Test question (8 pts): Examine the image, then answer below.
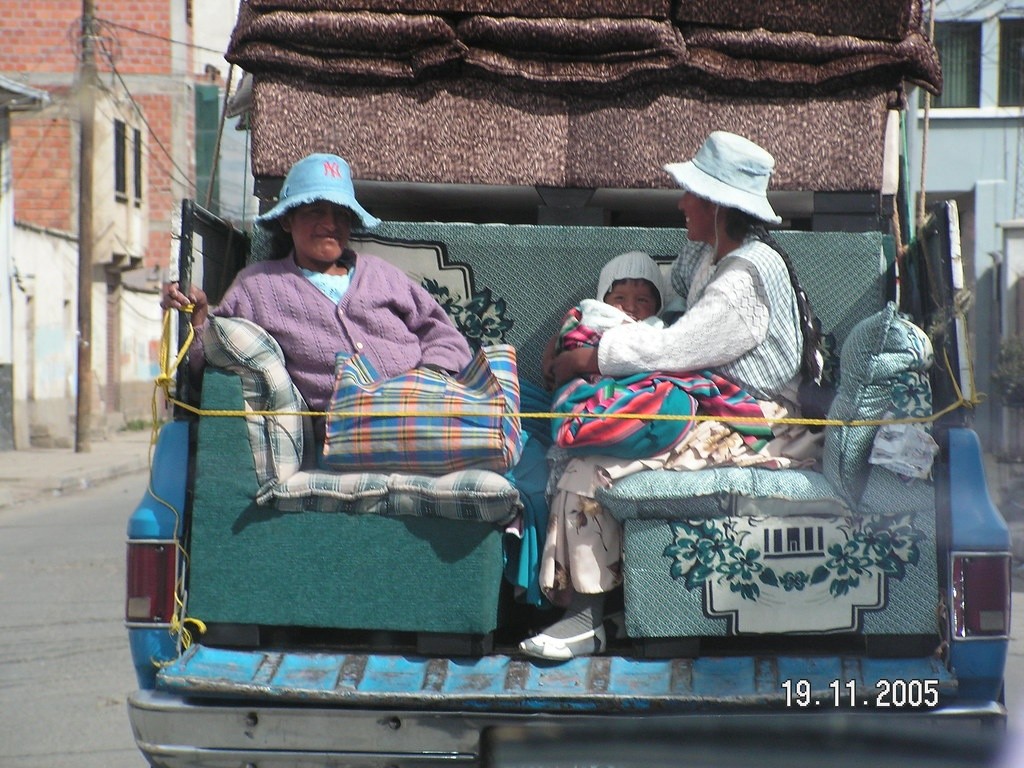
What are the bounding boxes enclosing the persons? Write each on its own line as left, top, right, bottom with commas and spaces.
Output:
160, 152, 567, 623
519, 130, 838, 661
550, 250, 699, 461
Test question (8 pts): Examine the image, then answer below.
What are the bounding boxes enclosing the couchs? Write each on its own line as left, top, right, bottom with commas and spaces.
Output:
592, 300, 943, 658
183, 311, 527, 657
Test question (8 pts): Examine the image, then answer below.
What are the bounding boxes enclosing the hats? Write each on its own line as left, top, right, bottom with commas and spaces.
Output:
663, 129, 784, 225
253, 153, 381, 233
596, 248, 668, 318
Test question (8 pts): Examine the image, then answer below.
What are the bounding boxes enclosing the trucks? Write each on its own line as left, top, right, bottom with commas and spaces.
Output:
125, 0, 1009, 768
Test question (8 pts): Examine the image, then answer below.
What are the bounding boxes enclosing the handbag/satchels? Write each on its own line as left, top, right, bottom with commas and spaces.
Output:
322, 343, 522, 476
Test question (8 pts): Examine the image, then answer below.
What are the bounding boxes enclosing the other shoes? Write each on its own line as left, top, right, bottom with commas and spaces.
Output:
527, 609, 629, 644
519, 625, 604, 661
